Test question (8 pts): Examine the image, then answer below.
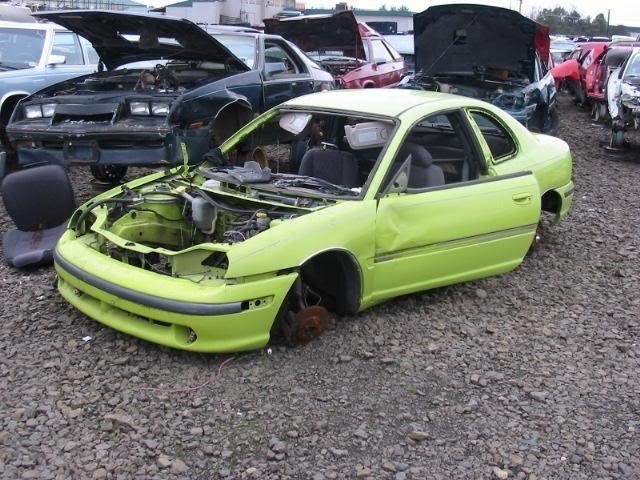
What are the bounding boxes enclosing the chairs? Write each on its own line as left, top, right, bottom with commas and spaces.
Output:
379, 141, 445, 193
2, 163, 78, 269
297, 148, 358, 190
